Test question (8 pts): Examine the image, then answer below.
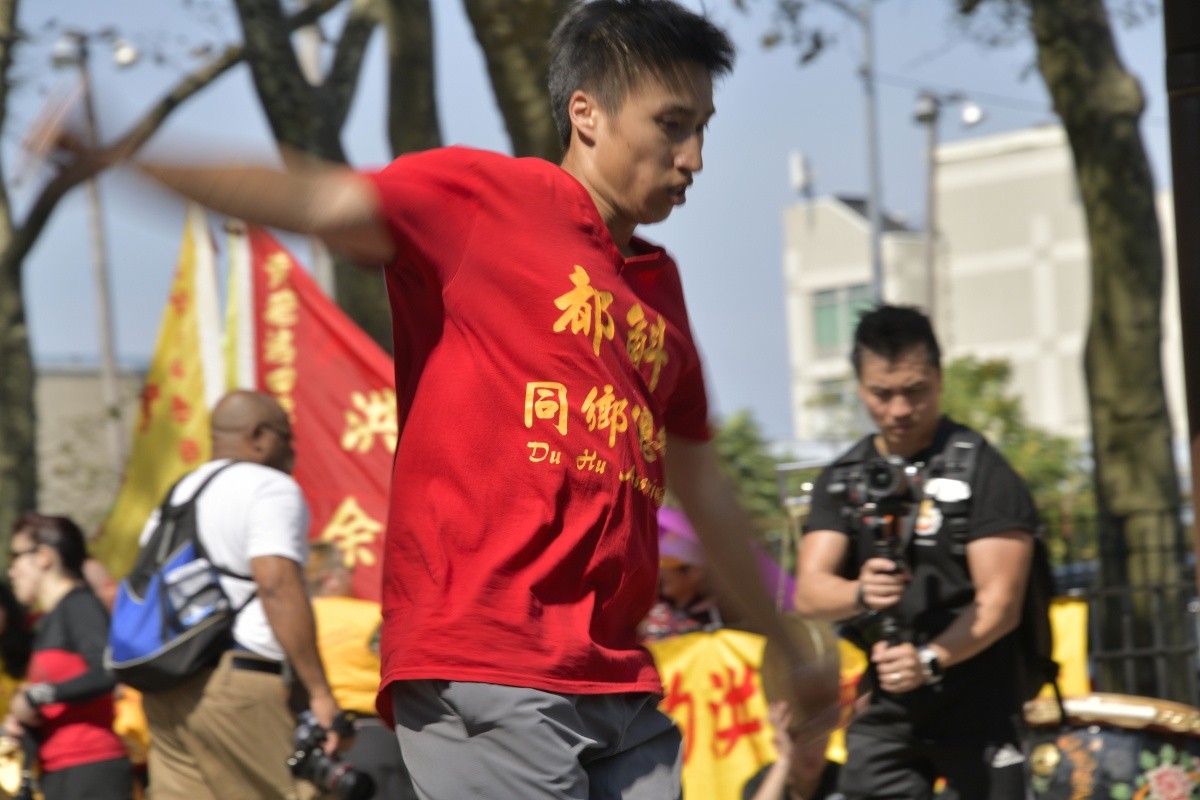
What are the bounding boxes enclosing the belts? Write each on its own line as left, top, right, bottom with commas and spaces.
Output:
204, 653, 283, 674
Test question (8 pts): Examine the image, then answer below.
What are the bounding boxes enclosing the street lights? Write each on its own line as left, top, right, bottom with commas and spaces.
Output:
910, 87, 985, 334
43, 26, 145, 477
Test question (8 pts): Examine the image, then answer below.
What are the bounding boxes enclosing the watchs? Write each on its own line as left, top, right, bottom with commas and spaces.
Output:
914, 644, 945, 687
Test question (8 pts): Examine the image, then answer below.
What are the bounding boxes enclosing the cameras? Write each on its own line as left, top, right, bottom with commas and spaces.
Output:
287, 723, 378, 800
846, 457, 922, 507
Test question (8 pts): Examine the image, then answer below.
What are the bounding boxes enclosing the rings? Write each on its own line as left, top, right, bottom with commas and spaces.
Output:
893, 673, 900, 682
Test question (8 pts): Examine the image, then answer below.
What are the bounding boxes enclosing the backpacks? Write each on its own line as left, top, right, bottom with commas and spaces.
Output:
102, 461, 256, 691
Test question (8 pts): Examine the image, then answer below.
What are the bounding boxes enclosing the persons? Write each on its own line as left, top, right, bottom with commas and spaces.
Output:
792, 307, 1043, 800
1, 516, 133, 800
128, 391, 356, 798
26, 0, 840, 800
